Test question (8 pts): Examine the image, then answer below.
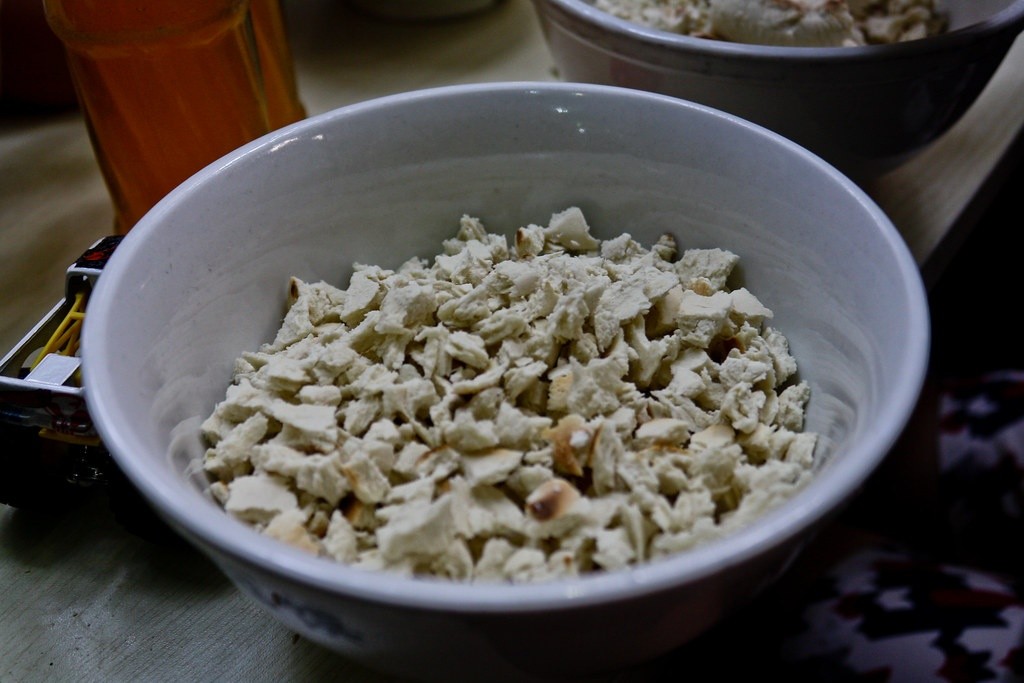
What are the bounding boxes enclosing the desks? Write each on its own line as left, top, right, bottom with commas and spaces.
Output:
0, 0, 1024, 683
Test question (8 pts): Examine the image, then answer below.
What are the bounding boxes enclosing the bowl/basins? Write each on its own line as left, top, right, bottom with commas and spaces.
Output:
523, 0, 1023, 178
82, 80, 930, 681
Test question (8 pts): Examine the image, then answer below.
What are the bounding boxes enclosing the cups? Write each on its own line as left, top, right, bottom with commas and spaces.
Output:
36, 0, 314, 268
